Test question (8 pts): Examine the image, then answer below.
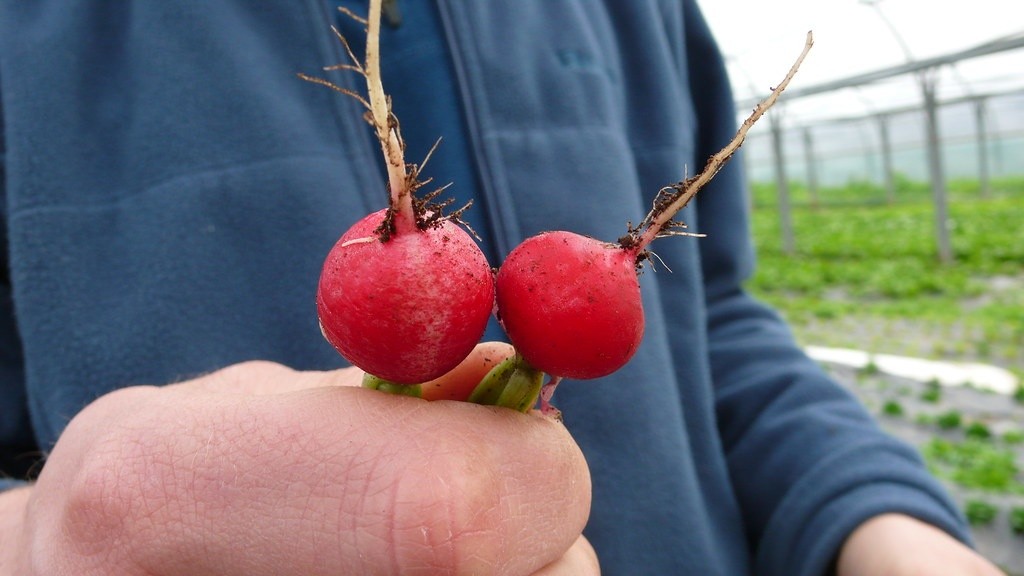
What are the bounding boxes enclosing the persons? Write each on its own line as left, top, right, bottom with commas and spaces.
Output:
1, 0, 1005, 576
